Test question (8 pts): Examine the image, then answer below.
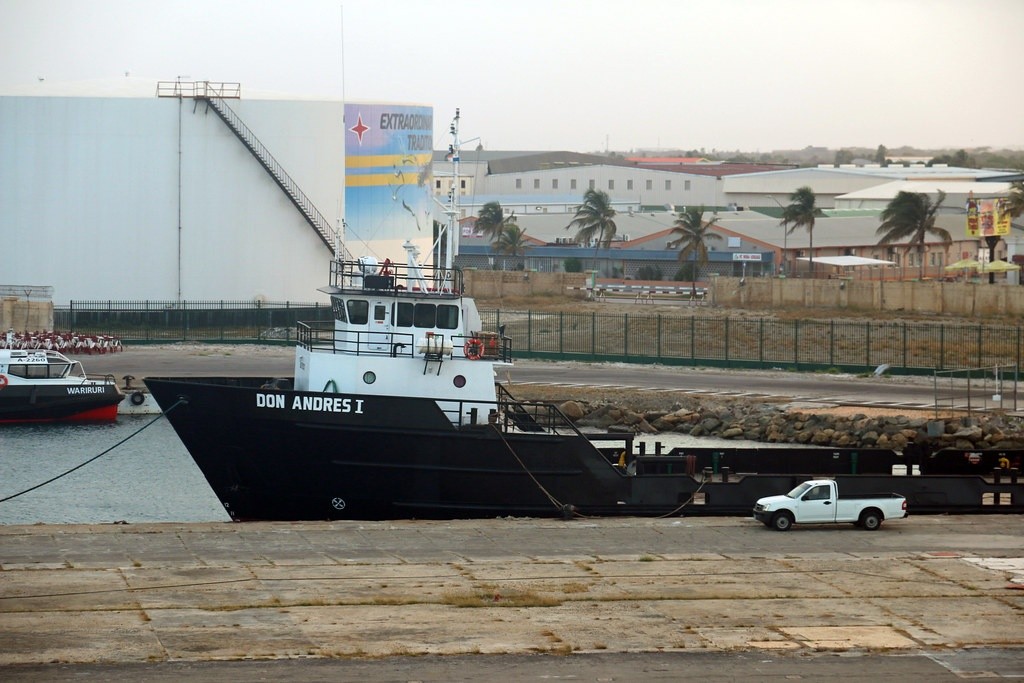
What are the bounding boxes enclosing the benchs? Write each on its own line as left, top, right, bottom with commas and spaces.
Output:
993, 467, 1018, 483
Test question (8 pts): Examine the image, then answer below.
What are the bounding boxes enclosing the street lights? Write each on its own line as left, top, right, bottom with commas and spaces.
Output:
766, 195, 787, 277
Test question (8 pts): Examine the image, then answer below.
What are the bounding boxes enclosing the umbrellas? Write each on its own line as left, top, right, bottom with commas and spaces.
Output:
977, 259, 1021, 285
946, 258, 980, 282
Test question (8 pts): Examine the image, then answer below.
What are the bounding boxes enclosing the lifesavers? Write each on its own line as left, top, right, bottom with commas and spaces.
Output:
0, 374, 8, 388
464, 339, 485, 360
131, 392, 144, 405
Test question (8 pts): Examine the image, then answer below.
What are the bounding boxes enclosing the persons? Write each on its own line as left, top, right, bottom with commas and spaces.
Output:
618, 451, 626, 473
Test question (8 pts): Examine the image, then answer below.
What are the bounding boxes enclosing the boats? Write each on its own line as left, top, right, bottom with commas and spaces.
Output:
0, 348, 126, 423
143, 103, 1024, 524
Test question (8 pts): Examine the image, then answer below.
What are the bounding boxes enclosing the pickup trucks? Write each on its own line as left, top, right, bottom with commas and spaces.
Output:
752, 479, 908, 532
